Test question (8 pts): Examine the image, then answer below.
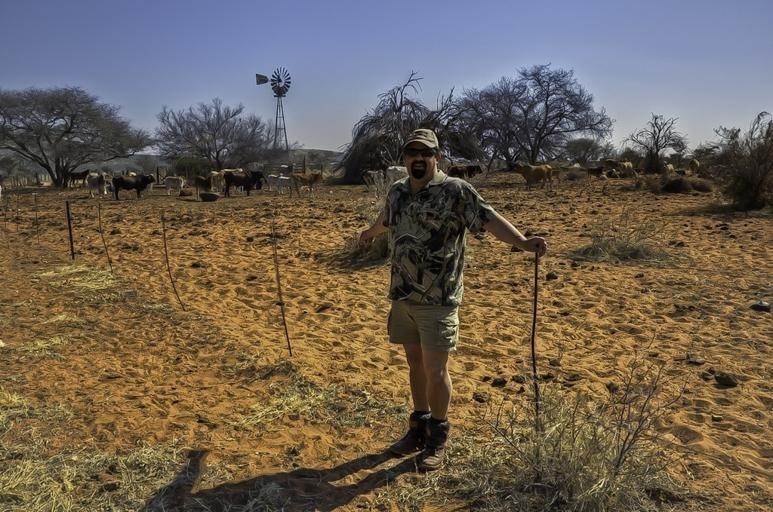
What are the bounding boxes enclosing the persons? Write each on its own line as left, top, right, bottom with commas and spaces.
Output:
356, 127, 549, 473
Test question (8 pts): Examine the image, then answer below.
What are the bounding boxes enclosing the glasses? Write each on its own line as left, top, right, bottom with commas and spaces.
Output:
404, 147, 437, 156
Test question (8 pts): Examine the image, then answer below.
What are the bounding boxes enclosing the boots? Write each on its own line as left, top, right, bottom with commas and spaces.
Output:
418, 421, 448, 470
390, 412, 431, 456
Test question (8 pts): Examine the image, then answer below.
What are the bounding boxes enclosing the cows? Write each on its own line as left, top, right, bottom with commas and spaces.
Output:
165, 176, 183, 196
664, 159, 699, 178
69, 169, 156, 200
360, 165, 409, 200
510, 160, 553, 192
193, 167, 263, 200
294, 167, 326, 200
588, 162, 649, 183
447, 165, 483, 181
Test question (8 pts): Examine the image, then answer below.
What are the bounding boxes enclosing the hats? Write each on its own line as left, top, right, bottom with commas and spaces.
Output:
403, 128, 440, 150
